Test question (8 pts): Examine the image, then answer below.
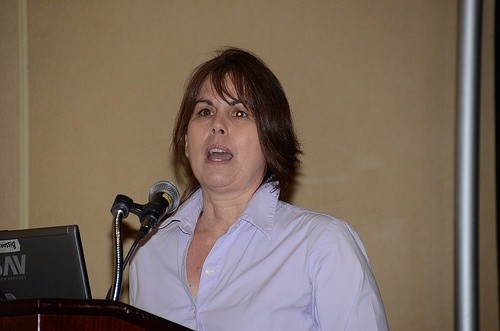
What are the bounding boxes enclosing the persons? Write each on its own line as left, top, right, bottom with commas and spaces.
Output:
129, 48, 390, 331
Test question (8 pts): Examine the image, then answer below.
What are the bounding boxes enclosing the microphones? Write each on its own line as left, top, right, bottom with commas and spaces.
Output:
139, 181, 180, 240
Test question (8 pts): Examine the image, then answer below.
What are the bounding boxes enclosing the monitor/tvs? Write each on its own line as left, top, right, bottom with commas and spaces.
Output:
0, 225, 92, 301
